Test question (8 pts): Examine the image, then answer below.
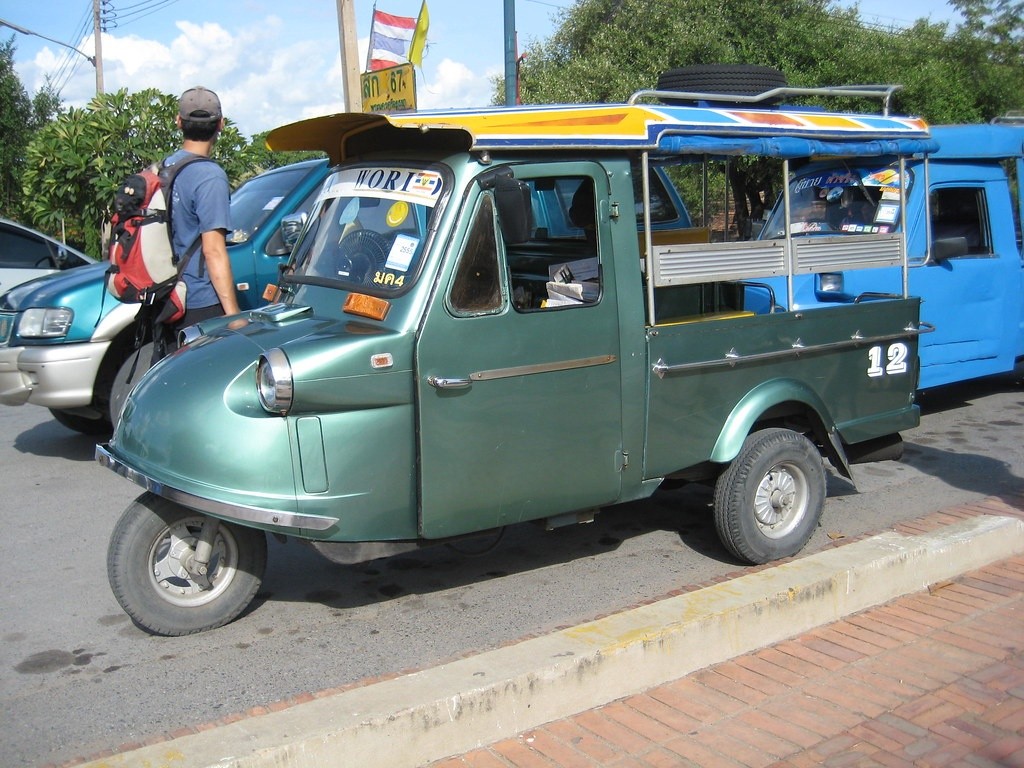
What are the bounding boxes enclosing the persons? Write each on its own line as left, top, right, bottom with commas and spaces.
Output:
152, 85, 249, 376
838, 187, 865, 224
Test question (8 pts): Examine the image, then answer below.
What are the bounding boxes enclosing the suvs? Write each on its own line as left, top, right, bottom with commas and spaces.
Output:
0, 157, 696, 434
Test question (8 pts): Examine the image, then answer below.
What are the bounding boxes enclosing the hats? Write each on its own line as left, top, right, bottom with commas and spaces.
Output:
180, 86, 222, 122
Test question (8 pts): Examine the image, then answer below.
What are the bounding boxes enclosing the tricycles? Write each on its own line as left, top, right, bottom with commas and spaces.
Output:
94, 65, 935, 636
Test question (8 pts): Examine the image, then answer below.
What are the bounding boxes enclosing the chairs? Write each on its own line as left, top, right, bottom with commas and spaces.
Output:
931, 201, 985, 257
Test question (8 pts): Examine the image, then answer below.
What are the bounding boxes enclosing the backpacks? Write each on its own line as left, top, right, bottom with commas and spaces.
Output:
108, 154, 216, 304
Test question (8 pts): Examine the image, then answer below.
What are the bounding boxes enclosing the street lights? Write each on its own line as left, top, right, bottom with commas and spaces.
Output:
0, 19, 104, 90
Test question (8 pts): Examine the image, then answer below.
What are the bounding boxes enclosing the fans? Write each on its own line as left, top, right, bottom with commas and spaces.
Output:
332, 229, 396, 290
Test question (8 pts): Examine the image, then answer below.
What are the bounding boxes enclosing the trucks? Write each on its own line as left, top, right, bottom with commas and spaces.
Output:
741, 117, 1024, 392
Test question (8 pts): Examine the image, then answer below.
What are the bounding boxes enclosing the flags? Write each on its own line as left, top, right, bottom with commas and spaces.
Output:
407, 0, 429, 68
369, 11, 417, 71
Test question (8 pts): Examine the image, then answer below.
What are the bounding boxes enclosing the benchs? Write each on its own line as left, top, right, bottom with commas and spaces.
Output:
636, 227, 785, 329
503, 244, 596, 296
